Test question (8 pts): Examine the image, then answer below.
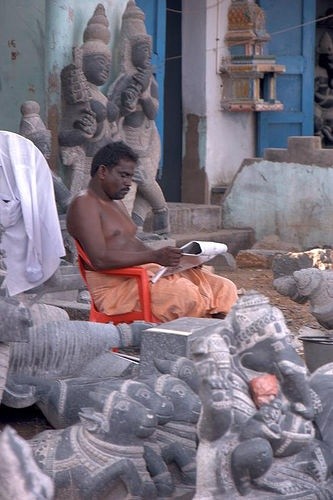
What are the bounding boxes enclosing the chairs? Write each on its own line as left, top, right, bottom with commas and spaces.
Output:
74, 239, 153, 324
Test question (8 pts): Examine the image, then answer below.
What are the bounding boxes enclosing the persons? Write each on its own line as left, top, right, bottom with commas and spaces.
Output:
66, 142, 240, 325
18, 1, 171, 265
